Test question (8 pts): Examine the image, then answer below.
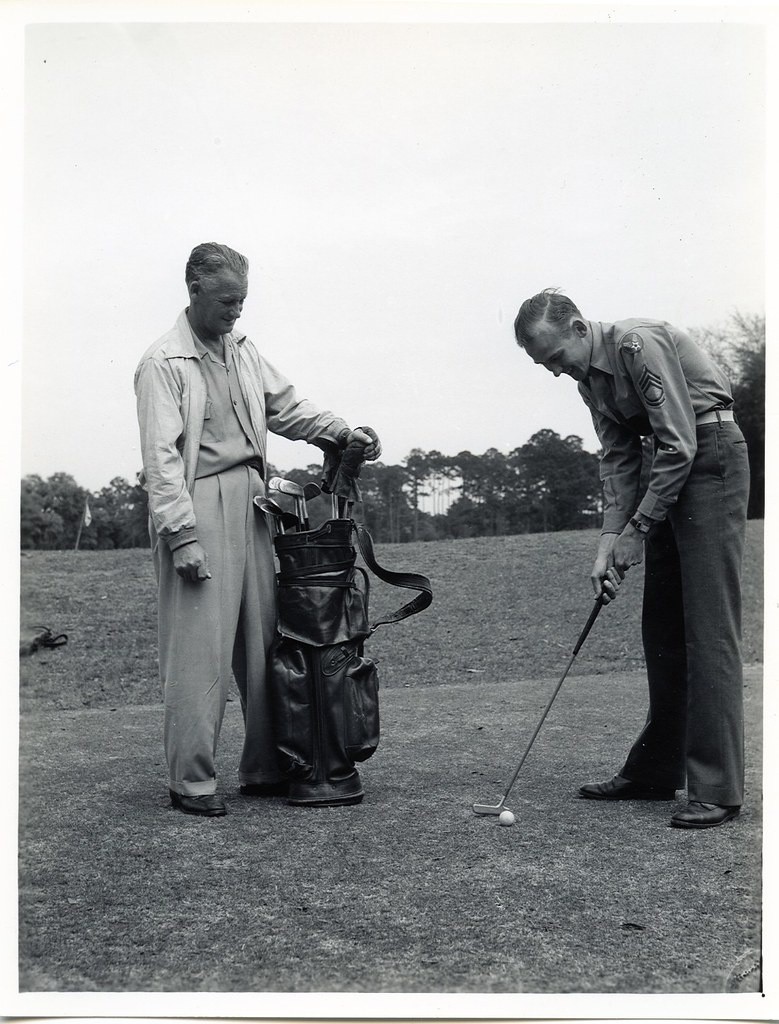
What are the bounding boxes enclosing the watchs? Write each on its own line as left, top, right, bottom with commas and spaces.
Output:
629, 518, 651, 534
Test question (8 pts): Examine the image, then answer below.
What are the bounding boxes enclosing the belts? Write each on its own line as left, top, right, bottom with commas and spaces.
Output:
695, 410, 733, 426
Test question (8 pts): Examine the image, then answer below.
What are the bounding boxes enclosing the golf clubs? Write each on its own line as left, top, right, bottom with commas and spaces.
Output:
472, 565, 618, 814
253, 434, 376, 538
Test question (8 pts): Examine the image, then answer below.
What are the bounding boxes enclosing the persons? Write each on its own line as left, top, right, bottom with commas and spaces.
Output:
133, 242, 381, 817
512, 290, 751, 831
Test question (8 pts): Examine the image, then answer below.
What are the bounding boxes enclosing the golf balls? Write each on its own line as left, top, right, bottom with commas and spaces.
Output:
499, 810, 515, 826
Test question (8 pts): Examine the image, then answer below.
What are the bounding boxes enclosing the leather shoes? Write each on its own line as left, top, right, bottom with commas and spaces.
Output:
240, 781, 285, 798
579, 775, 676, 800
169, 788, 225, 816
671, 802, 741, 828
